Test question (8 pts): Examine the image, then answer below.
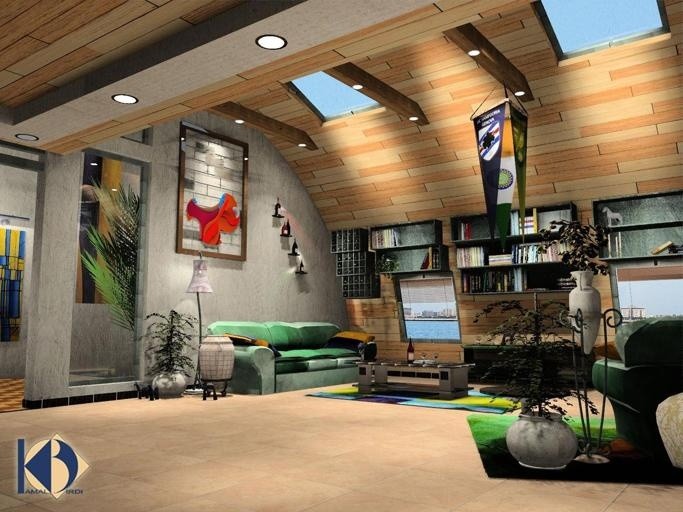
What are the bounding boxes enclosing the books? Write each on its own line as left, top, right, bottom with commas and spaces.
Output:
650, 240, 682, 256
369, 206, 573, 292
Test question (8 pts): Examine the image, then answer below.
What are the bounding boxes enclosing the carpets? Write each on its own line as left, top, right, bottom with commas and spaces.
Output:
465, 415, 677, 486
0, 375, 24, 412
303, 383, 521, 416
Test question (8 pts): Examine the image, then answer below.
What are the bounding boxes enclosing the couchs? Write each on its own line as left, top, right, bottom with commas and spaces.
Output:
207, 320, 378, 397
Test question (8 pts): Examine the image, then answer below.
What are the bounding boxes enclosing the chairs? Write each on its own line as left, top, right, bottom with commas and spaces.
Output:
591, 314, 683, 455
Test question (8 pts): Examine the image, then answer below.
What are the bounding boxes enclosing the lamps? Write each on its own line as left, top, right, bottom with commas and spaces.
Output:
183, 252, 215, 395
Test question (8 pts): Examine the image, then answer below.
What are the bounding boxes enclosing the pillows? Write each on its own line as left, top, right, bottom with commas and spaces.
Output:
327, 329, 375, 355
221, 331, 282, 358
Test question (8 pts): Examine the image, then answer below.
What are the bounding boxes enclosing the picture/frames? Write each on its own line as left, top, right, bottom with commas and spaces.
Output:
174, 119, 250, 262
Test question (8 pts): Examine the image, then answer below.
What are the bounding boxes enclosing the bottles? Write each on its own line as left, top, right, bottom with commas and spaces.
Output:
407, 338, 415, 363
282, 218, 290, 235
292, 237, 298, 254
299, 260, 304, 272
275, 197, 281, 216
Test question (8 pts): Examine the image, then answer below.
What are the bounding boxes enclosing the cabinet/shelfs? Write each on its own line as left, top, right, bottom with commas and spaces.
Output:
328, 217, 449, 301
592, 190, 682, 261
450, 201, 582, 294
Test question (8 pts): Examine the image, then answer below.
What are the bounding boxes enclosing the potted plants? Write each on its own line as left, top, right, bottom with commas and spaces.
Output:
532, 215, 610, 357
465, 293, 598, 473
134, 307, 201, 396
